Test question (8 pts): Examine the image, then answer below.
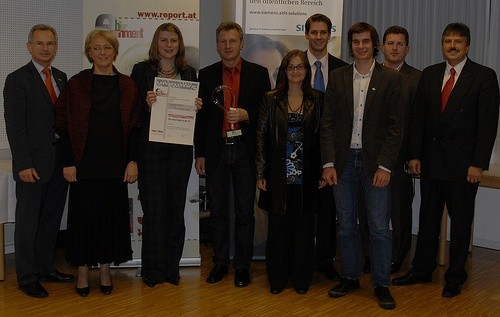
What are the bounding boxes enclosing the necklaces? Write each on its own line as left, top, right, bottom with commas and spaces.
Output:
157, 67, 179, 79
288, 103, 302, 113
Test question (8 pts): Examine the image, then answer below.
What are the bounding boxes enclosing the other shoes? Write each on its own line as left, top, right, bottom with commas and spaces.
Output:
389, 262, 399, 275
99, 273, 112, 294
363, 266, 371, 273
144, 277, 157, 287
165, 275, 179, 285
76, 274, 90, 297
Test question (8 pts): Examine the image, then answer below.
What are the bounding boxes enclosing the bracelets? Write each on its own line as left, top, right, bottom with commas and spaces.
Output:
130, 161, 137, 163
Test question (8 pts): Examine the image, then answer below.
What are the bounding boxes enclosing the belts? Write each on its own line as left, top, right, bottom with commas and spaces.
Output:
222, 137, 242, 145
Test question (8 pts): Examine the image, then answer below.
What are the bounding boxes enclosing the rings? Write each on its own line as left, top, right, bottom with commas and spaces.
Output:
135, 177, 137, 179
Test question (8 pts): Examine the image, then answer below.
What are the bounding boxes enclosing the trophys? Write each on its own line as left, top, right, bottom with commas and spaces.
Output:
212, 85, 242, 138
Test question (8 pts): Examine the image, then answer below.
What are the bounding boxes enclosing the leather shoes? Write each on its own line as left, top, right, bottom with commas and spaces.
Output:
391, 269, 433, 285
270, 285, 281, 294
206, 264, 228, 283
294, 281, 307, 294
234, 268, 251, 286
442, 282, 460, 298
328, 279, 360, 296
19, 279, 49, 298
39, 268, 74, 283
374, 286, 395, 309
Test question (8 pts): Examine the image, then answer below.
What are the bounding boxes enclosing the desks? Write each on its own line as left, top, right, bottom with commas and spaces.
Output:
0, 158, 15, 282
413, 166, 500, 265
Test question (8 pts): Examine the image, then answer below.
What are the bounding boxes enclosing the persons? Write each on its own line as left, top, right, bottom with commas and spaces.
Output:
362, 26, 422, 275
194, 21, 271, 287
130, 24, 197, 287
303, 14, 350, 281
241, 34, 289, 257
3, 24, 74, 298
392, 23, 500, 297
320, 22, 406, 310
56, 29, 138, 297
257, 49, 323, 296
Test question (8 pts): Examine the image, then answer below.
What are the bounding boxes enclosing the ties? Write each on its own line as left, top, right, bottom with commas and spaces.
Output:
228, 67, 237, 111
42, 68, 57, 106
313, 61, 325, 93
441, 68, 456, 112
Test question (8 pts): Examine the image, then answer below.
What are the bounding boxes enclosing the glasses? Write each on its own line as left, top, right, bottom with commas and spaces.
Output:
286, 63, 306, 71
